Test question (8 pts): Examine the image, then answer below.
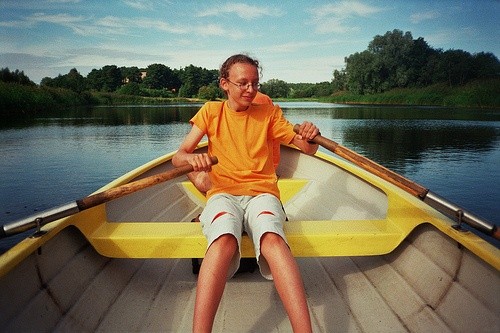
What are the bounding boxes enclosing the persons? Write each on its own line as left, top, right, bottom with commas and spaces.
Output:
171, 54, 322, 332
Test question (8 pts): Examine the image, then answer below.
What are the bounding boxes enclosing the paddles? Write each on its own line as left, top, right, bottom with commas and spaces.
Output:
0, 156, 218, 235
292, 124, 500, 240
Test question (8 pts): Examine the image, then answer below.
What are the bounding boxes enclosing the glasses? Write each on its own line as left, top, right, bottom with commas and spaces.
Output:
224, 78, 263, 90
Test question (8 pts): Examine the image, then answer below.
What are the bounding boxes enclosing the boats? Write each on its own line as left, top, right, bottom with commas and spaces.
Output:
0, 139, 499, 333
